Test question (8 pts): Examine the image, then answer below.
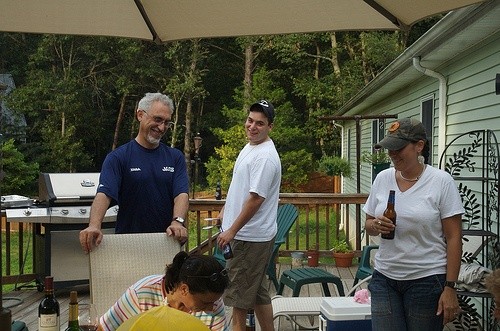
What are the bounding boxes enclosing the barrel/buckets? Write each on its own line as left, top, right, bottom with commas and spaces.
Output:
308, 252, 318, 266
290, 252, 304, 266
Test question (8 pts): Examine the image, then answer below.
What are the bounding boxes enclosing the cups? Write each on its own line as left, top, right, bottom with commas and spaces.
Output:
78, 303, 98, 331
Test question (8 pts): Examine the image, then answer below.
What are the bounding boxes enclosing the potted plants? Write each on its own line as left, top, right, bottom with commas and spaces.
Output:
333, 240, 355, 266
362, 150, 392, 172
317, 155, 354, 181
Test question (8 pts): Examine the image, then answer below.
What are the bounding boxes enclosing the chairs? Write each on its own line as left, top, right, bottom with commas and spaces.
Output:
350, 245, 382, 297
213, 204, 298, 293
271, 275, 374, 331
89, 232, 184, 327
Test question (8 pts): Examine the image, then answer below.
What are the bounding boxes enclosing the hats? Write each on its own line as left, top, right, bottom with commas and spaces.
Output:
250, 99, 274, 125
375, 118, 427, 150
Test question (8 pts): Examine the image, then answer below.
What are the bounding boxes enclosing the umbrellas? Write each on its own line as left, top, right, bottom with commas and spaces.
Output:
0, 0, 487, 43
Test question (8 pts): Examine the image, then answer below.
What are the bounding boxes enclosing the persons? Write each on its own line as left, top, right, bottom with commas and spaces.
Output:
360, 117, 466, 331
213, 99, 282, 331
79, 92, 189, 253
98, 252, 231, 330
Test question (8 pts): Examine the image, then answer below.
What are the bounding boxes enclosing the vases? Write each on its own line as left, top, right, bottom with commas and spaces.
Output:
307, 250, 318, 266
291, 252, 304, 268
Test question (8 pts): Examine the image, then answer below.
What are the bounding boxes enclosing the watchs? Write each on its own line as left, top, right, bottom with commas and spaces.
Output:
445, 281, 459, 290
172, 216, 184, 226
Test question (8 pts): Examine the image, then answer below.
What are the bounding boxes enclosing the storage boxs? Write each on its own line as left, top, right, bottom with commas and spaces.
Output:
319, 297, 374, 331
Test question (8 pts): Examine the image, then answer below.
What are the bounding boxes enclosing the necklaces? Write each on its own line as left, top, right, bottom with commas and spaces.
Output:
400, 162, 425, 183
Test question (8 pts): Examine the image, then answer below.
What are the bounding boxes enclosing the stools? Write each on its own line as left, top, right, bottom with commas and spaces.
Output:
277, 269, 345, 298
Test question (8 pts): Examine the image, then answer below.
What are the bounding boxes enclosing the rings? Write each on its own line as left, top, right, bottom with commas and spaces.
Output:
378, 221, 382, 226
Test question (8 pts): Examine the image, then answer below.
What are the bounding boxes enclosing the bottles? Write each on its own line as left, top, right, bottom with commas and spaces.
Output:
64, 291, 85, 331
216, 223, 233, 260
38, 276, 60, 331
216, 182, 221, 200
380, 190, 397, 240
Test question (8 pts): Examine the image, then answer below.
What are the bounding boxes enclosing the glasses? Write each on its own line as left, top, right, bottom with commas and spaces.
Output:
141, 109, 174, 129
186, 268, 229, 282
